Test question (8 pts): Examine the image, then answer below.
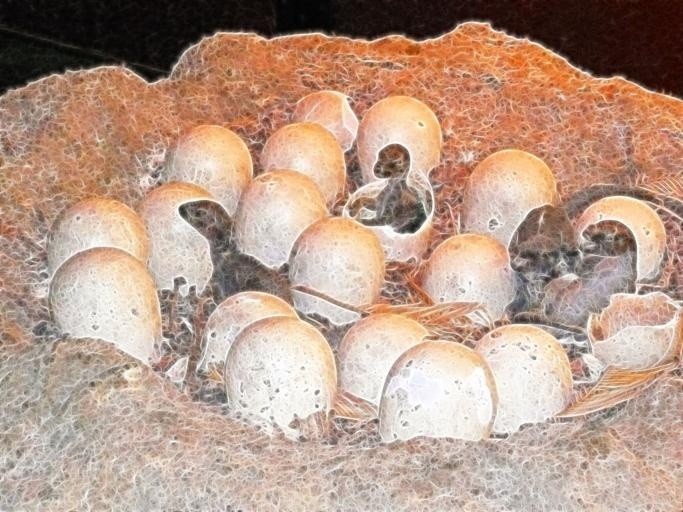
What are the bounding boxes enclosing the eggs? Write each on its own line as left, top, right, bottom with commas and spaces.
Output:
44, 87, 667, 442
